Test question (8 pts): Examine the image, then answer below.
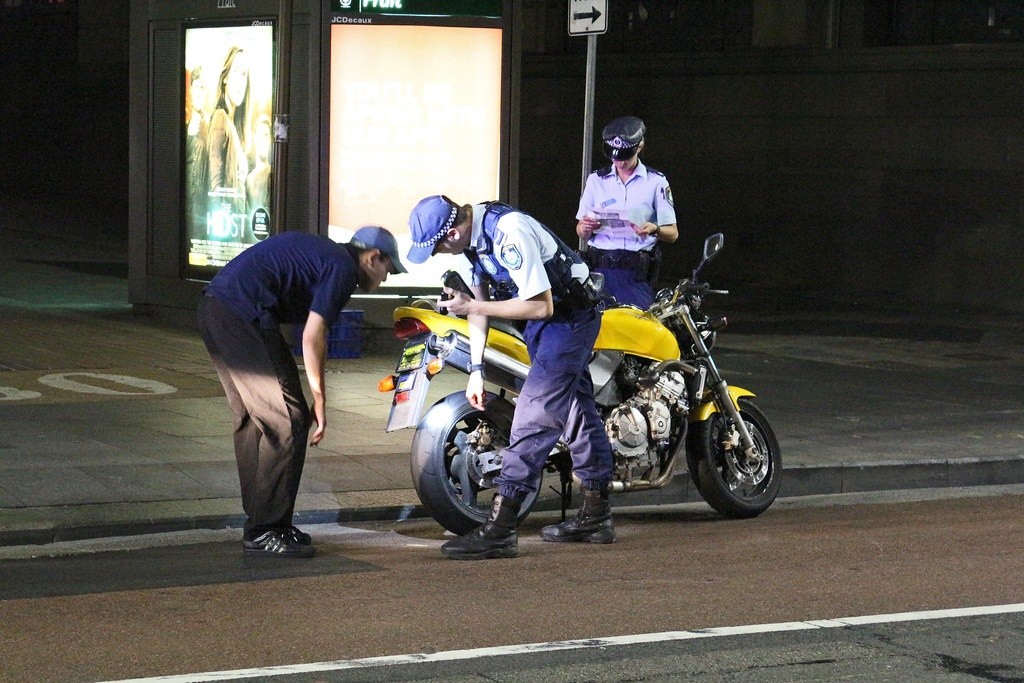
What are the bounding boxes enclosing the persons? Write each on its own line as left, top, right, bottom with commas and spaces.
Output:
576, 116, 681, 317
409, 196, 615, 557
185, 44, 273, 245
196, 224, 396, 556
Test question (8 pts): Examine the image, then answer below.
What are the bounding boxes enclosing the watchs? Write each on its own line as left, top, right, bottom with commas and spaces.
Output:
467, 360, 486, 376
647, 223, 662, 236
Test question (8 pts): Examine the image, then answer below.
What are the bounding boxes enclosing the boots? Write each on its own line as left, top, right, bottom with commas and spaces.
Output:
440, 493, 522, 558
539, 485, 616, 543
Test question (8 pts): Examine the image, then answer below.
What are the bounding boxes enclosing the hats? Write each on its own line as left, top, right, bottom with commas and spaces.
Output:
406, 194, 457, 265
602, 115, 646, 162
352, 225, 407, 274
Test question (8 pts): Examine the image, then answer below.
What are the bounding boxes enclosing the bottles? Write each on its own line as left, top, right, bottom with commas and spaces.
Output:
441, 270, 476, 299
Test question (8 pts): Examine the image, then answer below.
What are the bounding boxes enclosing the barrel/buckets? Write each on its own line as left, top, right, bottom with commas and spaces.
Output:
328, 311, 363, 359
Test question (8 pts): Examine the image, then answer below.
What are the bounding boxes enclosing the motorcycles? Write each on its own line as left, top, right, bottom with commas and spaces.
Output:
377, 232, 783, 536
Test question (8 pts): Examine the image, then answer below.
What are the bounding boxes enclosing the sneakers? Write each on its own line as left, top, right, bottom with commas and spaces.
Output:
241, 527, 317, 559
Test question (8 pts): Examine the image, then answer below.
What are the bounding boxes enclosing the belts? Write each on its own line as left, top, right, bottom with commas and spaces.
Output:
585, 253, 656, 270
